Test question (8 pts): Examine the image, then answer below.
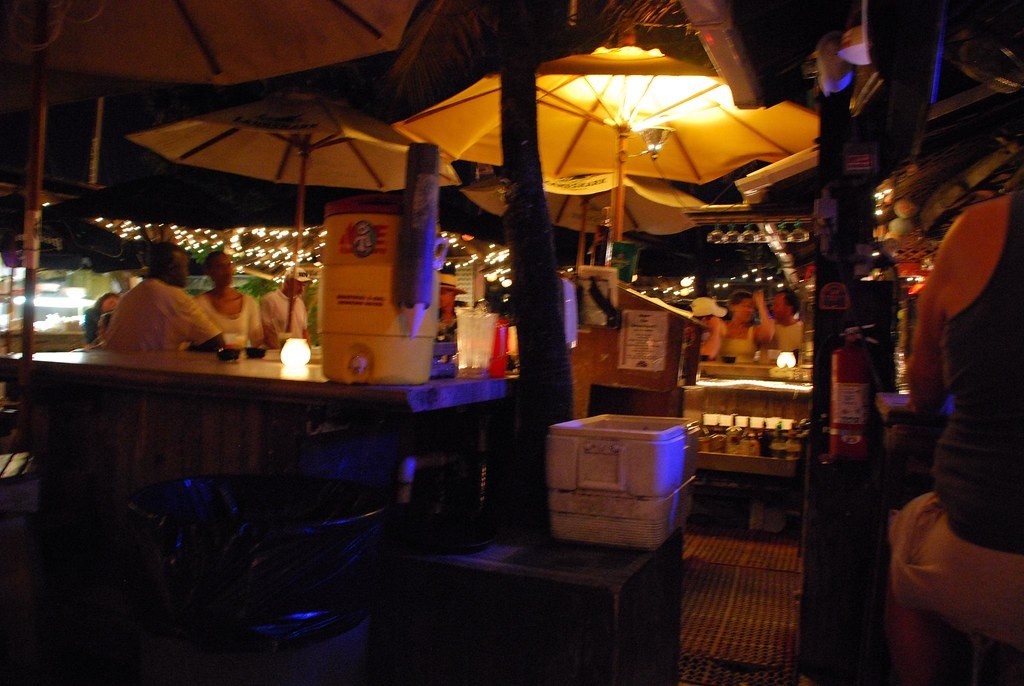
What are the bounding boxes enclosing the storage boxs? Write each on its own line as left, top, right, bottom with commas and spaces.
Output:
548, 413, 698, 498
550, 480, 692, 548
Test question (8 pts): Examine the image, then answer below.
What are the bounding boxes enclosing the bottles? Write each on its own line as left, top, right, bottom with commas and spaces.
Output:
725, 415, 800, 461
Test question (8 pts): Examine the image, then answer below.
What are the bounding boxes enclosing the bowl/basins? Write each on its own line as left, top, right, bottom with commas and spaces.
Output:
218, 347, 239, 361
245, 346, 266, 359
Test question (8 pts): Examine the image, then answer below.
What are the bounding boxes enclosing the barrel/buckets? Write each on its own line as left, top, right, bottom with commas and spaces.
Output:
321, 194, 438, 383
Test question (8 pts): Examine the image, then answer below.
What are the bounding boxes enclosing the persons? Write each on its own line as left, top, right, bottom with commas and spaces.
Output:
691, 289, 775, 362
193, 251, 266, 347
768, 288, 803, 352
85, 293, 120, 343
888, 164, 1024, 686
260, 267, 311, 348
101, 241, 225, 352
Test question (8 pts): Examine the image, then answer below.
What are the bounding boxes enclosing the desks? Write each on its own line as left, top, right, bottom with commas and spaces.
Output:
392, 530, 684, 686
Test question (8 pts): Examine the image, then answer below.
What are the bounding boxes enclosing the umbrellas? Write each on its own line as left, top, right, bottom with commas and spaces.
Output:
125, 85, 461, 341
52, 168, 261, 239
392, 47, 820, 240
0, 0, 419, 84
0, 188, 142, 273
461, 171, 707, 266
